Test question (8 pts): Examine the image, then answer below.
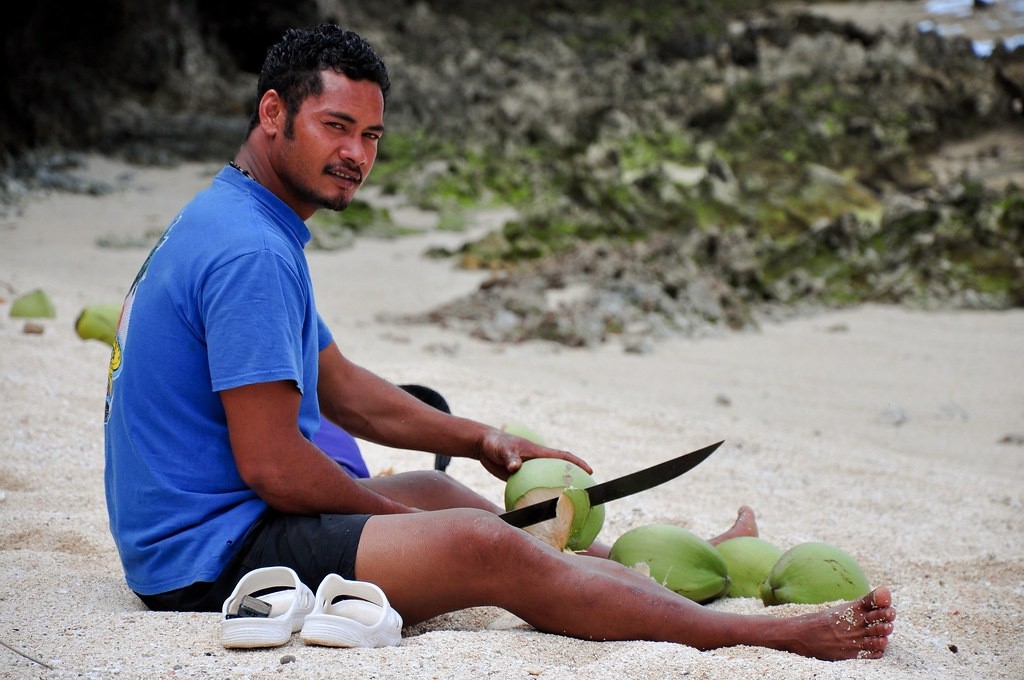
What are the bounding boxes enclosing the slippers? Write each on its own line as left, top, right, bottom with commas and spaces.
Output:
301, 573, 405, 649
220, 566, 314, 649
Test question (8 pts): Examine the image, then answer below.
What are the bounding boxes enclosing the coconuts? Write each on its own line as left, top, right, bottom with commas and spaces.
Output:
499, 423, 872, 605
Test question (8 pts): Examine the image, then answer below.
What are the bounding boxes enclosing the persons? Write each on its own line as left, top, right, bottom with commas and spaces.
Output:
102, 19, 894, 666
